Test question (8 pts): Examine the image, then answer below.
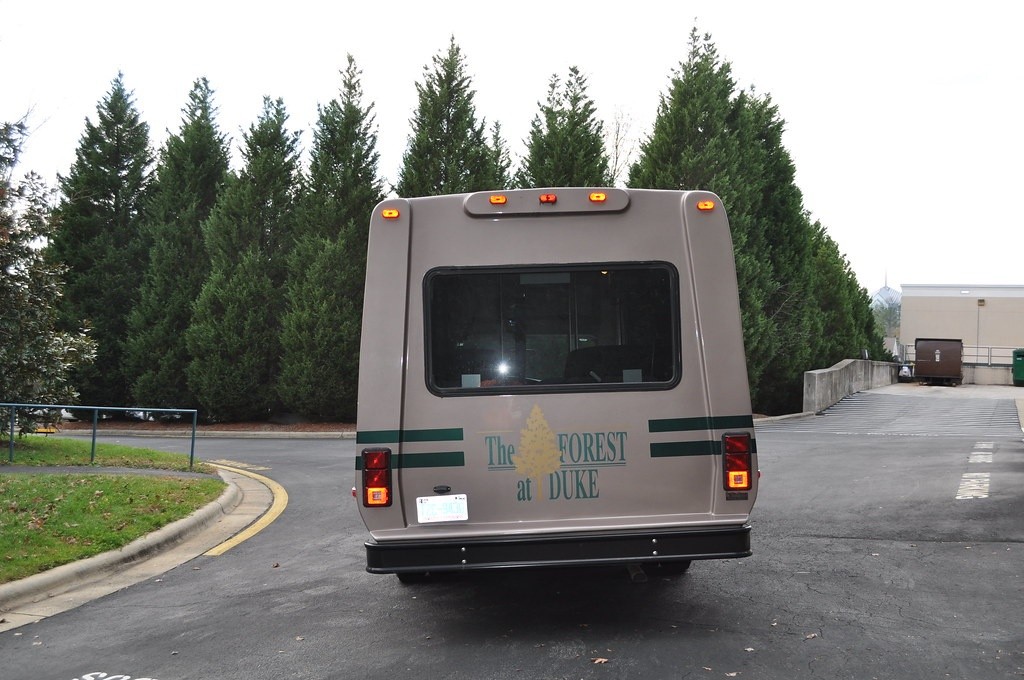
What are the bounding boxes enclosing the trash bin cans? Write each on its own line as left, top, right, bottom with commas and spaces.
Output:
913, 337, 963, 387
1011, 348, 1024, 386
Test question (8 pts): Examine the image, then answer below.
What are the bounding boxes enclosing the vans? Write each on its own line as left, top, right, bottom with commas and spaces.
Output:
351, 187, 764, 588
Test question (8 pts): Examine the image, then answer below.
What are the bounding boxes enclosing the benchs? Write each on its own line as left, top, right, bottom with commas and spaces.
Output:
423, 301, 655, 388
